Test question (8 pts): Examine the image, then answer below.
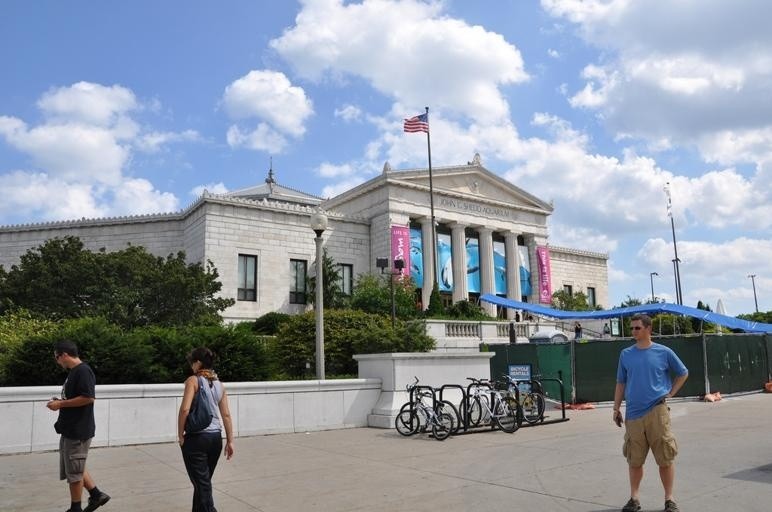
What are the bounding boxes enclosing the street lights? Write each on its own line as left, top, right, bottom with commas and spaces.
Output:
375, 256, 407, 329
308, 208, 332, 379
650, 272, 660, 303
747, 275, 759, 314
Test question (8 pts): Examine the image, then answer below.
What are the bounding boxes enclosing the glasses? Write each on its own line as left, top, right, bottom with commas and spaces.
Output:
55, 354, 60, 361
630, 327, 641, 330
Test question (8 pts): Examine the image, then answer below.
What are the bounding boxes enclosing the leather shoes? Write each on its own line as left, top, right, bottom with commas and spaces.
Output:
83, 492, 110, 512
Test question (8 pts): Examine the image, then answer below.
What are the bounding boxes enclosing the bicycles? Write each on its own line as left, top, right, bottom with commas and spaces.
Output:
394, 374, 546, 440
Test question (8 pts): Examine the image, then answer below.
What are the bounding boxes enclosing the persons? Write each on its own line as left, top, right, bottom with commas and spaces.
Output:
45, 337, 111, 512
602, 323, 610, 335
612, 315, 688, 512
574, 321, 581, 339
176, 348, 234, 511
515, 311, 520, 321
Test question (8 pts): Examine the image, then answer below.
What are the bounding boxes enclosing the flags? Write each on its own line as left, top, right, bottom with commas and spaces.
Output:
402, 112, 428, 134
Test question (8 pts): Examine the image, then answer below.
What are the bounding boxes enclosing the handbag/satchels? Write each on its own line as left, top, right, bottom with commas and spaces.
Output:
184, 373, 213, 433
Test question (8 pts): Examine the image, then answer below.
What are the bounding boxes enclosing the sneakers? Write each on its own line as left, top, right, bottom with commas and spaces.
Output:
665, 500, 679, 512
622, 500, 641, 512
